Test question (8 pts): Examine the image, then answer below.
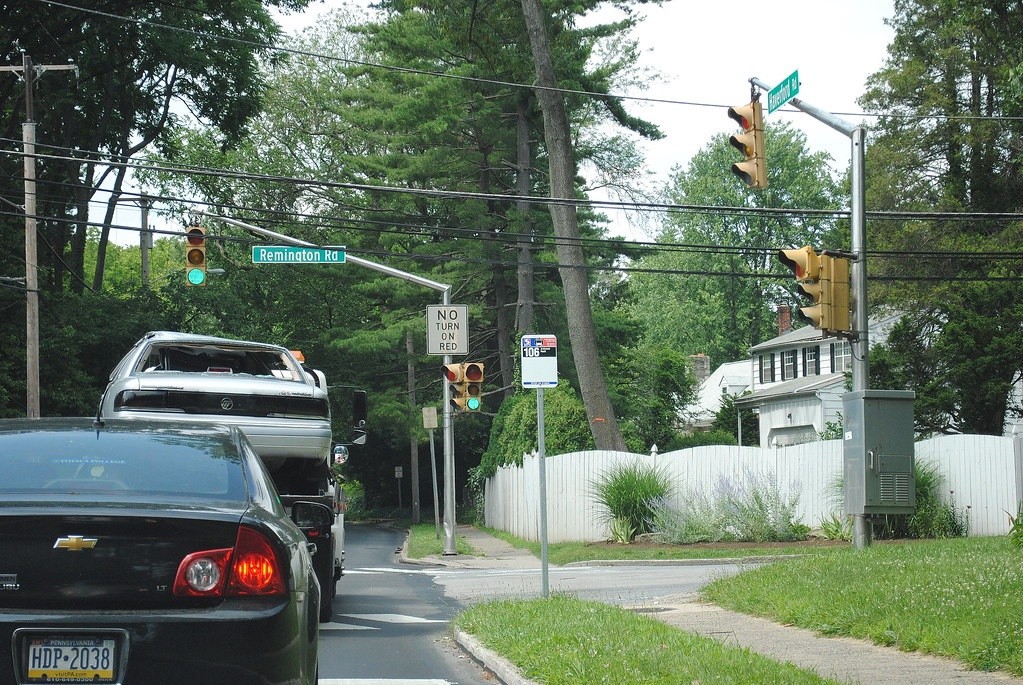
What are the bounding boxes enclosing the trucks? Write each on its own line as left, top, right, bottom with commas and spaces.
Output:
271, 350, 366, 622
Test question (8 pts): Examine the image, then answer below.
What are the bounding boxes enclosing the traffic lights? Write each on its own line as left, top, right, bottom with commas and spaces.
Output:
440, 363, 466, 411
727, 102, 768, 190
185, 226, 206, 287
463, 363, 484, 412
778, 246, 850, 332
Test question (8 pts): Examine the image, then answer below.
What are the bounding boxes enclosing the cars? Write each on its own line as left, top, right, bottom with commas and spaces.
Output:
0, 395, 321, 685
98, 332, 332, 484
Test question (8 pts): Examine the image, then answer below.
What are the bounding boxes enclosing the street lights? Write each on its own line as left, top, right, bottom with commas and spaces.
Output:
150, 268, 226, 284
